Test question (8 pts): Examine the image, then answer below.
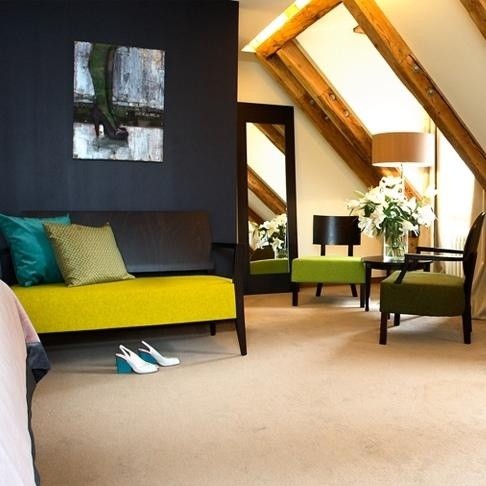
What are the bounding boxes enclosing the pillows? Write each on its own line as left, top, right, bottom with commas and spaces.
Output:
42, 221, 136, 287
0, 211, 70, 288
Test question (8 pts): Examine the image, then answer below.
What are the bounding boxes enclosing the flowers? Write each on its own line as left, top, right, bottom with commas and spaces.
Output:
258, 216, 289, 255
343, 174, 439, 257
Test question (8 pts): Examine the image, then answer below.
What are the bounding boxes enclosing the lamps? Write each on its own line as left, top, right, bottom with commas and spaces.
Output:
371, 132, 436, 204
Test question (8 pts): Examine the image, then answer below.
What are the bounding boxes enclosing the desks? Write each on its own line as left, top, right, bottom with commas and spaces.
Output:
361, 254, 433, 320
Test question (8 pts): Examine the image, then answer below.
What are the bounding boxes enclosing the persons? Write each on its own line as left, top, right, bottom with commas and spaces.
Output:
87, 43, 129, 141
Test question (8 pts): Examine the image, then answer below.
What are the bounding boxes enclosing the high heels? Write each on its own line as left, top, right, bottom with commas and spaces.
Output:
138, 340, 180, 367
117, 123, 127, 131
91, 105, 128, 140
115, 344, 160, 374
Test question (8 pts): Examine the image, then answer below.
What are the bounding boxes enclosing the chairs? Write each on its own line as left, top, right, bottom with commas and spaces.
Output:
291, 214, 366, 306
379, 212, 486, 345
250, 258, 289, 275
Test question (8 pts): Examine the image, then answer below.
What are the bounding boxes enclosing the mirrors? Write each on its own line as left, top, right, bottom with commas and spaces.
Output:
245, 121, 290, 275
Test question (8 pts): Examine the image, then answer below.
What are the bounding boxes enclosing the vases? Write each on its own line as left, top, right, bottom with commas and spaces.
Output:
274, 239, 287, 259
383, 228, 408, 261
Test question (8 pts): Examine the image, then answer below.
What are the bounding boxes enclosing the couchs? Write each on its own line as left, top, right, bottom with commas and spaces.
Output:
0, 207, 248, 355
